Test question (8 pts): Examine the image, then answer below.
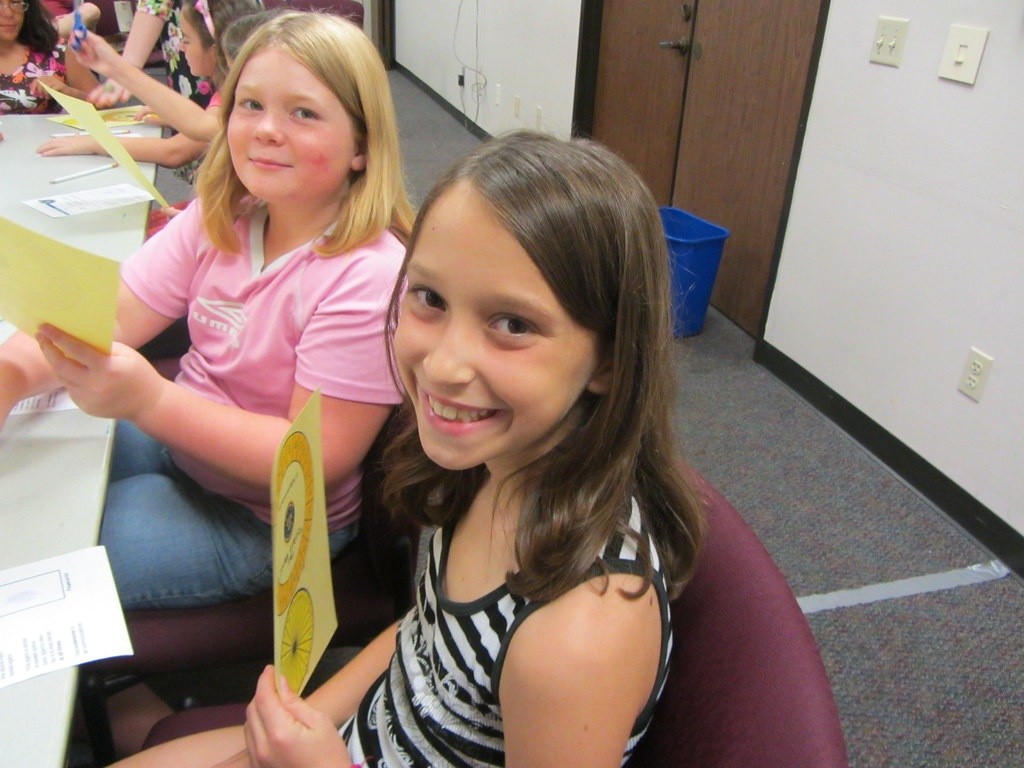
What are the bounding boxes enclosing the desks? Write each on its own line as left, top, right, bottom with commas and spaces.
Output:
0, 104, 160, 768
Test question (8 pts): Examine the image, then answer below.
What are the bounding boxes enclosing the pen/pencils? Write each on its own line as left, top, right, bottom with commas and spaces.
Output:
48, 162, 118, 184
48, 130, 130, 138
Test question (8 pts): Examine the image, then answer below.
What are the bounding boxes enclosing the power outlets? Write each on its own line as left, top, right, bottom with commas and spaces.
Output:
956, 345, 994, 401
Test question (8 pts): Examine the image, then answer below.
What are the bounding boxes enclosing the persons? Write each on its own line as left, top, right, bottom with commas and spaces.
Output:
0, 0, 301, 243
103, 130, 710, 768
0, 15, 418, 752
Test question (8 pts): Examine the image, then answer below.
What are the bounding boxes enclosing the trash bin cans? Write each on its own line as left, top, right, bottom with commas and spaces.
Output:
656, 205, 729, 338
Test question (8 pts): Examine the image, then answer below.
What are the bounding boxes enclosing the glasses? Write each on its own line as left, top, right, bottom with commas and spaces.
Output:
0, 0, 29, 13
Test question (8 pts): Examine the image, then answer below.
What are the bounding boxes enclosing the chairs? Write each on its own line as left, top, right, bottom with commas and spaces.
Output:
83, 404, 849, 768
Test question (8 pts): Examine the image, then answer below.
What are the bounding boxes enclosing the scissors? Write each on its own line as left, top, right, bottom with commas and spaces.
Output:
71, 0, 87, 49
